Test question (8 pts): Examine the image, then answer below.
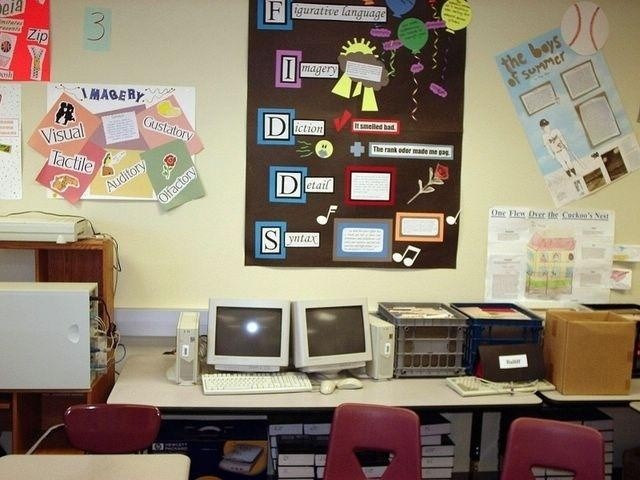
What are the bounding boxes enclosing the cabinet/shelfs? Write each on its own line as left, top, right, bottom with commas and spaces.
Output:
0, 238, 115, 453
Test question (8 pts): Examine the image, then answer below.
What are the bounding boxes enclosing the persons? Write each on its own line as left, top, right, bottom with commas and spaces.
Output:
539, 118, 576, 178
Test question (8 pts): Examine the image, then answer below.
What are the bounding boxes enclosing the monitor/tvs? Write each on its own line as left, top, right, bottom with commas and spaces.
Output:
206, 297, 291, 373
290, 296, 372, 385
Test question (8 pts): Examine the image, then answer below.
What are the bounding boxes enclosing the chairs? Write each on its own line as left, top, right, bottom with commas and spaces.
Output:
22, 402, 161, 454
319, 403, 425, 480
501, 415, 607, 480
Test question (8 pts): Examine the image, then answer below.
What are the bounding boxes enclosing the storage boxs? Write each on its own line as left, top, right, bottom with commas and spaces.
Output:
544, 309, 637, 395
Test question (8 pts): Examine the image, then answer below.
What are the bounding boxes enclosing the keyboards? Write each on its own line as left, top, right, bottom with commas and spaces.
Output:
444, 376, 556, 398
201, 370, 313, 395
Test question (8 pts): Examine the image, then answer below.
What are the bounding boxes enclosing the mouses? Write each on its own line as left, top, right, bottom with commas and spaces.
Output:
320, 379, 335, 395
336, 377, 363, 390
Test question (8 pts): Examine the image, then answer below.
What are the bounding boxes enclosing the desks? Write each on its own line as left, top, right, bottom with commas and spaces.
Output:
106, 354, 541, 480
0, 450, 192, 480
534, 372, 640, 415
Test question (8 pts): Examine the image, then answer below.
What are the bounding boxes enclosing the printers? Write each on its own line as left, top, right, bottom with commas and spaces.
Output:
0, 214, 88, 244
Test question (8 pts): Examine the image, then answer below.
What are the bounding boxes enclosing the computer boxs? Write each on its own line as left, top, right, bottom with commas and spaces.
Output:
176, 312, 200, 386
0, 281, 99, 390
365, 313, 395, 383
